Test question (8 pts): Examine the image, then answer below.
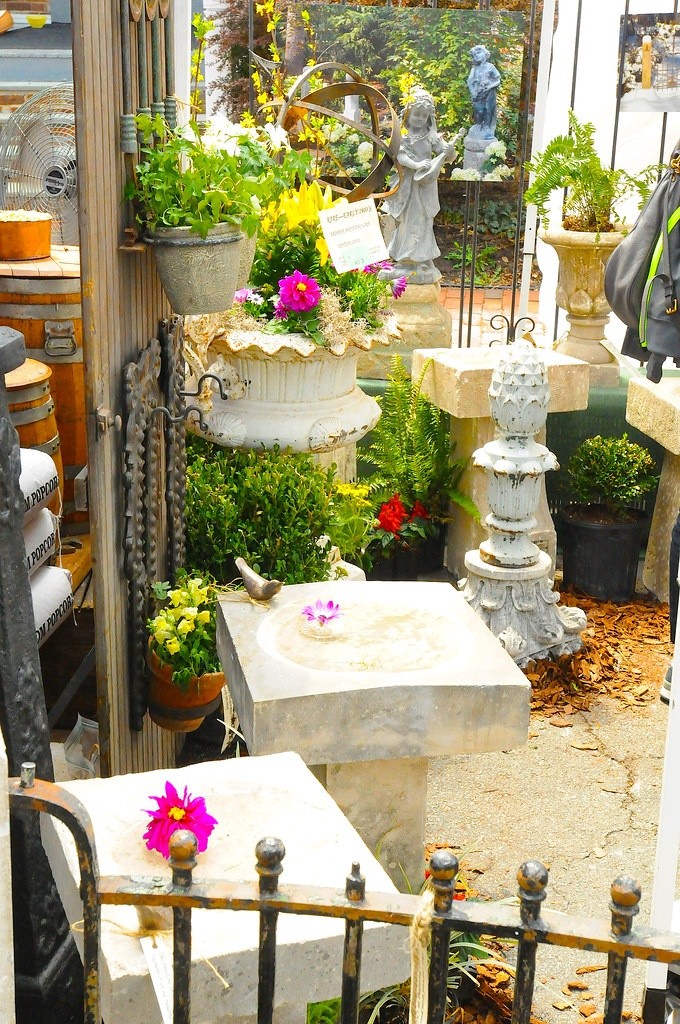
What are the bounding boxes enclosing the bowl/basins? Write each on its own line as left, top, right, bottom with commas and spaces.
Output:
1, 218, 53, 261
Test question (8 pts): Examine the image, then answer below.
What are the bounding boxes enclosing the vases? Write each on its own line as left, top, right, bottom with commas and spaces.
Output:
142, 219, 245, 313
148, 635, 226, 734
185, 316, 383, 491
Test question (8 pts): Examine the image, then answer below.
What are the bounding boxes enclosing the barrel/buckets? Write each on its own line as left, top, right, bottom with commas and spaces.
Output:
0, 277, 86, 515
3, 381, 63, 566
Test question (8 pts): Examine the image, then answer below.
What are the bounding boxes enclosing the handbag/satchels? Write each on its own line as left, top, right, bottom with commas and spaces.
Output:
604, 168, 680, 357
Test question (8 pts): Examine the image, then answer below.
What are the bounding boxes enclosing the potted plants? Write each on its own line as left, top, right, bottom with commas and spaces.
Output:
522, 104, 669, 365
556, 430, 659, 603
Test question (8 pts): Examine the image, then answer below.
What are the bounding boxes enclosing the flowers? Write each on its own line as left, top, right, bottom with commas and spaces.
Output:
142, 567, 233, 687
233, 257, 407, 347
377, 497, 440, 544
145, 785, 220, 865
307, 117, 379, 178
451, 138, 516, 183
116, 104, 314, 243
301, 595, 344, 631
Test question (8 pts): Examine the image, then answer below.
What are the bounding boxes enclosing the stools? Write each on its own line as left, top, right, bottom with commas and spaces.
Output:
216, 581, 530, 894
40, 751, 414, 1024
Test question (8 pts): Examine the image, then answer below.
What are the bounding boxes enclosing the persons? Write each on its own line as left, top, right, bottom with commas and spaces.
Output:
382, 90, 458, 270
466, 45, 502, 140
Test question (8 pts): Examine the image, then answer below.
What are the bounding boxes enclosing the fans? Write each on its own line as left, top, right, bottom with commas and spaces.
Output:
0, 83, 80, 248
